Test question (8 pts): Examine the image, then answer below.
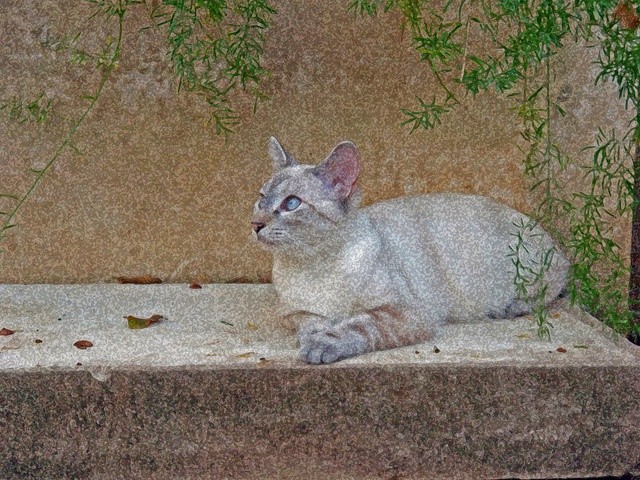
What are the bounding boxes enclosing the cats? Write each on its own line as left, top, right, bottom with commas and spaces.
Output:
251, 136, 571, 365
